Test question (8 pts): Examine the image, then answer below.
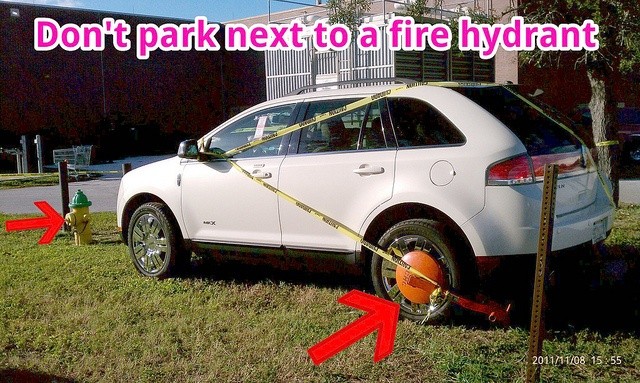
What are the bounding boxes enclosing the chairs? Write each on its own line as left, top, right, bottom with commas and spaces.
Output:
312, 115, 351, 153
363, 117, 386, 148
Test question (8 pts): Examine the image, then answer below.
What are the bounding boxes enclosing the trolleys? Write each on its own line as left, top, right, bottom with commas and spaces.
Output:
53, 144, 93, 180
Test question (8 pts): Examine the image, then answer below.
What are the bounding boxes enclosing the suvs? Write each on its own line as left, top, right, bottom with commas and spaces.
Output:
570, 106, 640, 163
116, 77, 617, 323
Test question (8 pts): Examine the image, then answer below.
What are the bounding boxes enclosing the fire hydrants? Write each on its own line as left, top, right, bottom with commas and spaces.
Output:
64, 188, 93, 245
